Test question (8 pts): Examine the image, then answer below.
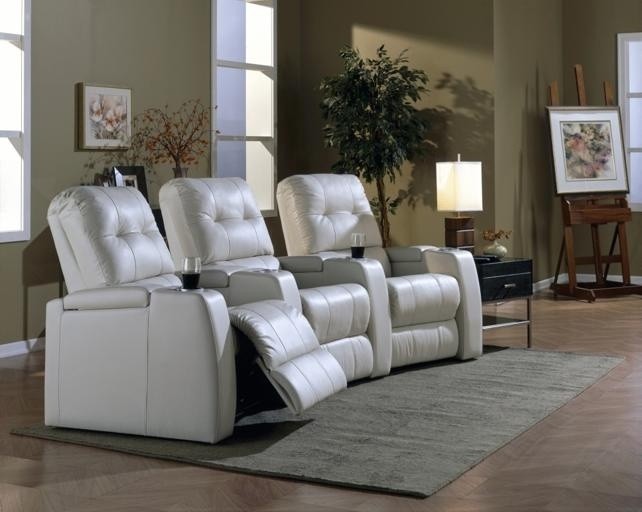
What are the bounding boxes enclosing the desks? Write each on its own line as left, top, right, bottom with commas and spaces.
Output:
473, 257, 533, 350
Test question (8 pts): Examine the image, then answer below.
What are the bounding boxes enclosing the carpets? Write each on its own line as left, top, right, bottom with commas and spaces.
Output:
13, 344, 622, 501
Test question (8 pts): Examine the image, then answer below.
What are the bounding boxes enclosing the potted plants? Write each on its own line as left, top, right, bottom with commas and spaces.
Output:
81, 101, 220, 178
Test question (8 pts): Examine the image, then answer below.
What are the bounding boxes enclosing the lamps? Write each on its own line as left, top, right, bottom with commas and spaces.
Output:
433, 153, 483, 254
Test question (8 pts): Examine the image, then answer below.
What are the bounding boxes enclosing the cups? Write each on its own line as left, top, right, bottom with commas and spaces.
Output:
350, 233, 367, 259
182, 256, 202, 290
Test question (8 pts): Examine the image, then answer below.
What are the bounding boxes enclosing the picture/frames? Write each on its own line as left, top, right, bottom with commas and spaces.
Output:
547, 103, 628, 195
75, 82, 134, 151
118, 165, 149, 200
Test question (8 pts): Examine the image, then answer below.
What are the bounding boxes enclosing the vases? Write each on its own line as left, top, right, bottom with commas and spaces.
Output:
485, 242, 507, 259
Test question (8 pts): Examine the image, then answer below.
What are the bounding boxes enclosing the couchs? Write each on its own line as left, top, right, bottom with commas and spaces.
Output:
158, 172, 394, 399
41, 173, 346, 442
272, 173, 482, 380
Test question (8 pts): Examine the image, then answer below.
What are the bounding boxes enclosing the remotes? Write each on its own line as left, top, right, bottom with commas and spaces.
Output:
475, 254, 498, 259
475, 257, 489, 261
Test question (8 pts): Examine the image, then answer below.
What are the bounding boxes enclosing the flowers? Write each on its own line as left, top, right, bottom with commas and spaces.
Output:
480, 229, 511, 243
